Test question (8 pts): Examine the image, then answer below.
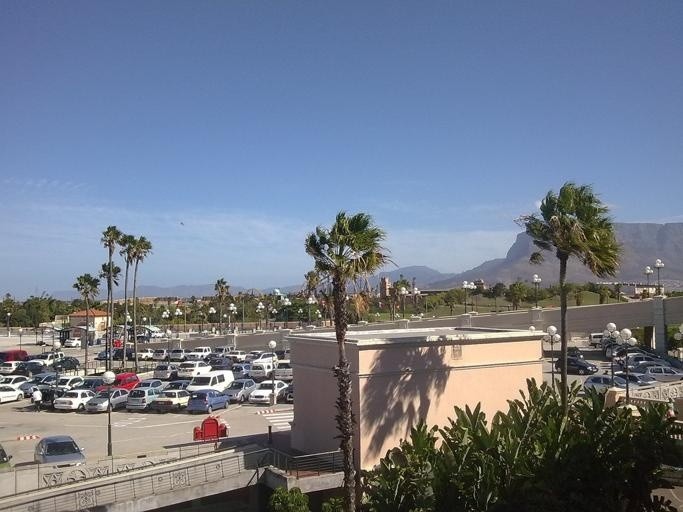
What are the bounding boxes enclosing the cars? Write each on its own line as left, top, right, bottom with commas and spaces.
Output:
553, 332, 682, 398
0, 331, 294, 413
33, 436, 86, 472
0, 444, 13, 474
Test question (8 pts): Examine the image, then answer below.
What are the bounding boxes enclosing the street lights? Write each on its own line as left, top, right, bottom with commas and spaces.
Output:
231, 310, 237, 330
222, 314, 227, 330
143, 317, 146, 342
615, 328, 637, 404
655, 258, 664, 296
126, 316, 131, 341
227, 303, 236, 330
602, 323, 619, 388
166, 310, 169, 330
374, 286, 423, 323
468, 282, 476, 311
255, 293, 321, 330
530, 274, 541, 308
542, 326, 560, 390
102, 371, 116, 456
162, 312, 168, 334
208, 306, 216, 332
7, 312, 10, 332
18, 329, 23, 350
175, 309, 182, 333
643, 265, 653, 297
461, 279, 470, 313
